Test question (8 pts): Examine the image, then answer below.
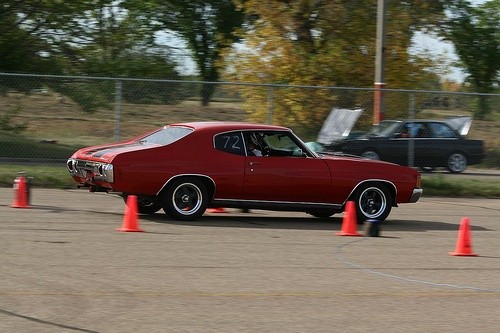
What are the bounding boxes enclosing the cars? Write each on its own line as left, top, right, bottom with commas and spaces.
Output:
313, 104, 489, 174
65, 120, 423, 226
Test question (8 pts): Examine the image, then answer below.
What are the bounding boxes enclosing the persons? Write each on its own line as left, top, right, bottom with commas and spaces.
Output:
246, 138, 271, 156
399, 127, 408, 138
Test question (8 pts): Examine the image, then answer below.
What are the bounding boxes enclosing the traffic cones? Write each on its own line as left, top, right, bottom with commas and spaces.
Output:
206, 207, 228, 213
334, 201, 365, 237
114, 195, 146, 232
10, 175, 33, 209
447, 216, 479, 256
